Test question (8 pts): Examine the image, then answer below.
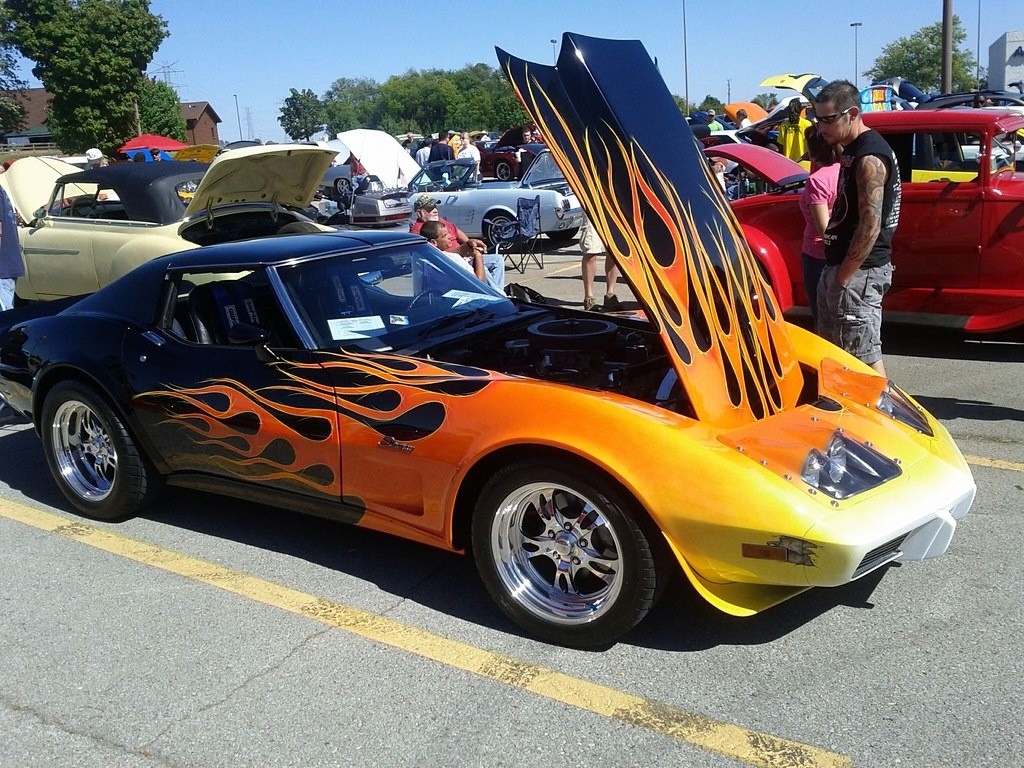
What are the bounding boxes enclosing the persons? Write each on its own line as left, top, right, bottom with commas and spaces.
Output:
1, 183, 24, 410
891, 100, 896, 110
410, 193, 506, 298
814, 78, 902, 379
579, 209, 625, 312
328, 128, 544, 190
703, 98, 818, 200
800, 125, 842, 315
86, 146, 168, 169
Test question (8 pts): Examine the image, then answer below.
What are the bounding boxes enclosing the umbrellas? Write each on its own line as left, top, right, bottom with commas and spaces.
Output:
117, 133, 190, 152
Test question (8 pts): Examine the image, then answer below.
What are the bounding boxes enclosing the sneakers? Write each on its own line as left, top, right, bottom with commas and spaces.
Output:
603, 294, 623, 311
583, 296, 599, 312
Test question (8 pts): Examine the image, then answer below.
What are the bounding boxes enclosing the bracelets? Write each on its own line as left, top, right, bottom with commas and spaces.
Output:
466, 240, 473, 249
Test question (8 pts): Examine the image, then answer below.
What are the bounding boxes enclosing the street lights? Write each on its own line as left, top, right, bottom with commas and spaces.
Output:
233, 95, 242, 142
550, 39, 556, 67
851, 23, 862, 87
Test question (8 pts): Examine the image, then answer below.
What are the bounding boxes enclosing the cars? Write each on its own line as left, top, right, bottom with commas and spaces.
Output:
697, 72, 1024, 180
0, 142, 386, 303
702, 109, 1023, 333
42, 125, 585, 251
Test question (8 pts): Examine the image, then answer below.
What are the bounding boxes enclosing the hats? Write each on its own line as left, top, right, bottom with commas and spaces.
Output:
150, 148, 160, 154
86, 148, 102, 164
707, 109, 715, 116
479, 130, 488, 135
414, 193, 441, 208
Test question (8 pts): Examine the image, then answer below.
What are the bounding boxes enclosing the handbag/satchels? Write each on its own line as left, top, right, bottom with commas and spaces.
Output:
504, 282, 548, 306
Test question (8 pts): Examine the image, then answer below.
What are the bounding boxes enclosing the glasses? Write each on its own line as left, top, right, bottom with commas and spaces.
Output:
815, 108, 851, 123
460, 137, 468, 140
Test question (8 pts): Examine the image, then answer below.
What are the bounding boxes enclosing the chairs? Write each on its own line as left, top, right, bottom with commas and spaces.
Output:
293, 260, 372, 337
185, 280, 283, 348
482, 195, 544, 275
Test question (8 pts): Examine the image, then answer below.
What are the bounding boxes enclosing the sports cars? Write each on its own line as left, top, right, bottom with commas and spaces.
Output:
0, 32, 977, 646
